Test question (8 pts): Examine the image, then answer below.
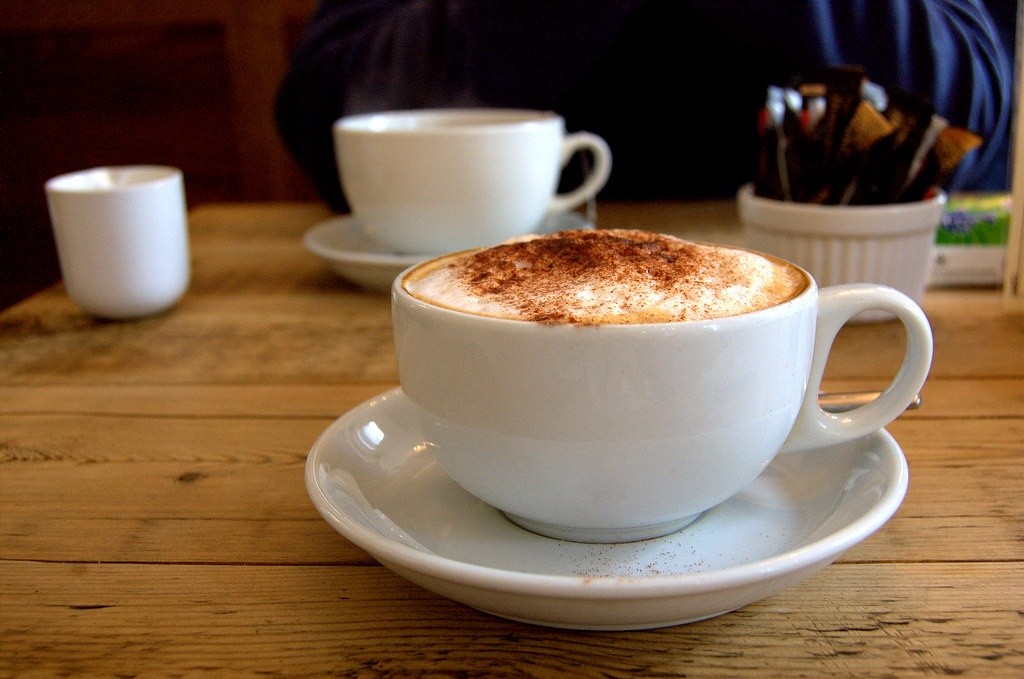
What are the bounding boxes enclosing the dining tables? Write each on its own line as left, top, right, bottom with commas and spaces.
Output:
0, 199, 1024, 678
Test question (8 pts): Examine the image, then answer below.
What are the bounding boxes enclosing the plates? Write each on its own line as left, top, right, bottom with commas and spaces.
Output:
304, 384, 909, 631
302, 210, 597, 296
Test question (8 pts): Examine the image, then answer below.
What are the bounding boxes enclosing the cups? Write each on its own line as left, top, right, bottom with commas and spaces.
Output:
735, 180, 948, 324
44, 165, 192, 320
334, 108, 613, 256
390, 227, 933, 545
